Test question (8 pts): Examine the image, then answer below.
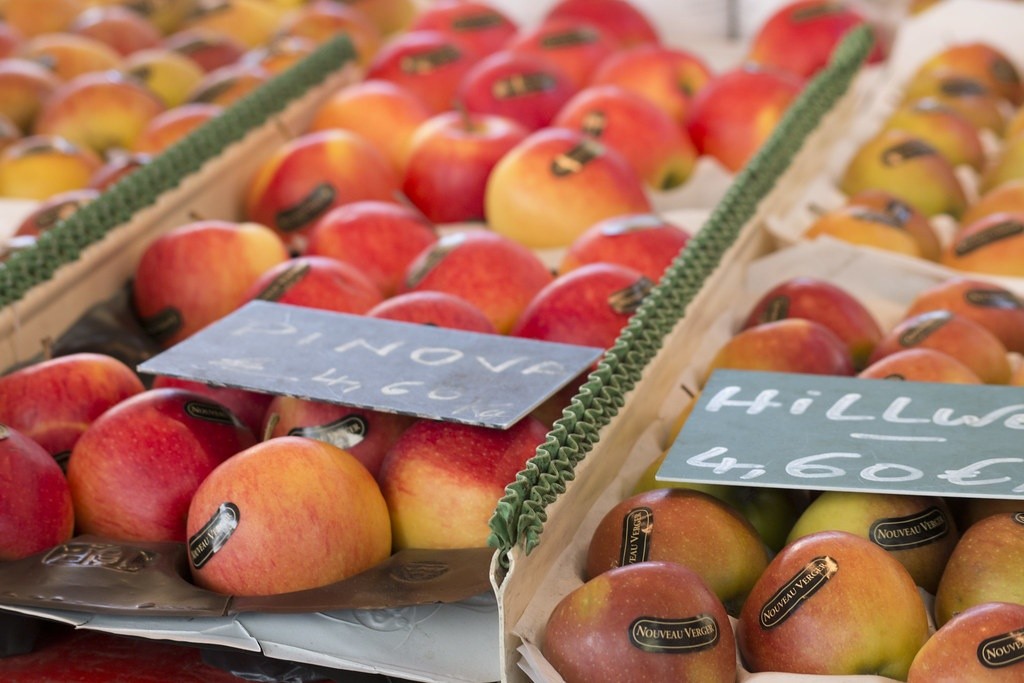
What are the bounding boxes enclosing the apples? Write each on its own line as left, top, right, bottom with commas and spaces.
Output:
0, 0, 897, 597
0, 625, 251, 683
531, 47, 1024, 683
